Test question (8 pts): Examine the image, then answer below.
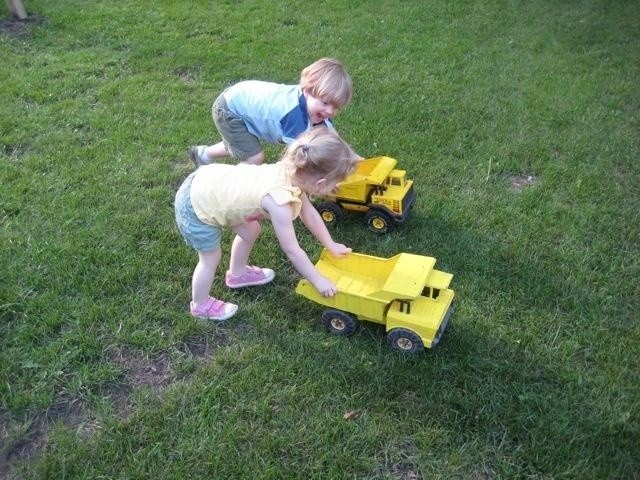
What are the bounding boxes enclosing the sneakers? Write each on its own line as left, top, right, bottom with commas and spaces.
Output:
224, 264, 278, 291
187, 294, 240, 321
188, 144, 216, 170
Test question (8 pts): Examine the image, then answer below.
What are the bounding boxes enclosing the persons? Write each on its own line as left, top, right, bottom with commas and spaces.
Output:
186, 57, 367, 198
173, 123, 353, 322
